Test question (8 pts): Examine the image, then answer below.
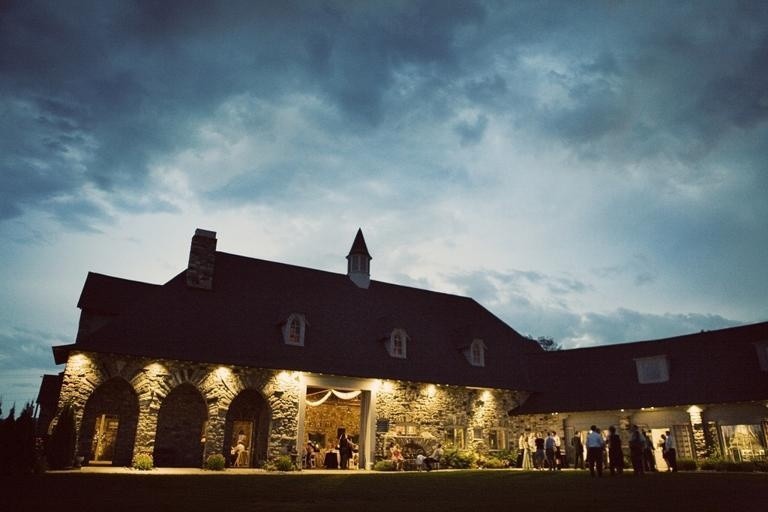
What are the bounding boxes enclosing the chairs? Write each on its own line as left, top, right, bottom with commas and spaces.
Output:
290, 452, 440, 472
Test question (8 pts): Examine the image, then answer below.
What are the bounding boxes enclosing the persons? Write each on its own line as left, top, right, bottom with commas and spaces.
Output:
230, 430, 248, 467
389, 441, 405, 471
521, 423, 678, 480
424, 443, 444, 472
281, 432, 358, 470
